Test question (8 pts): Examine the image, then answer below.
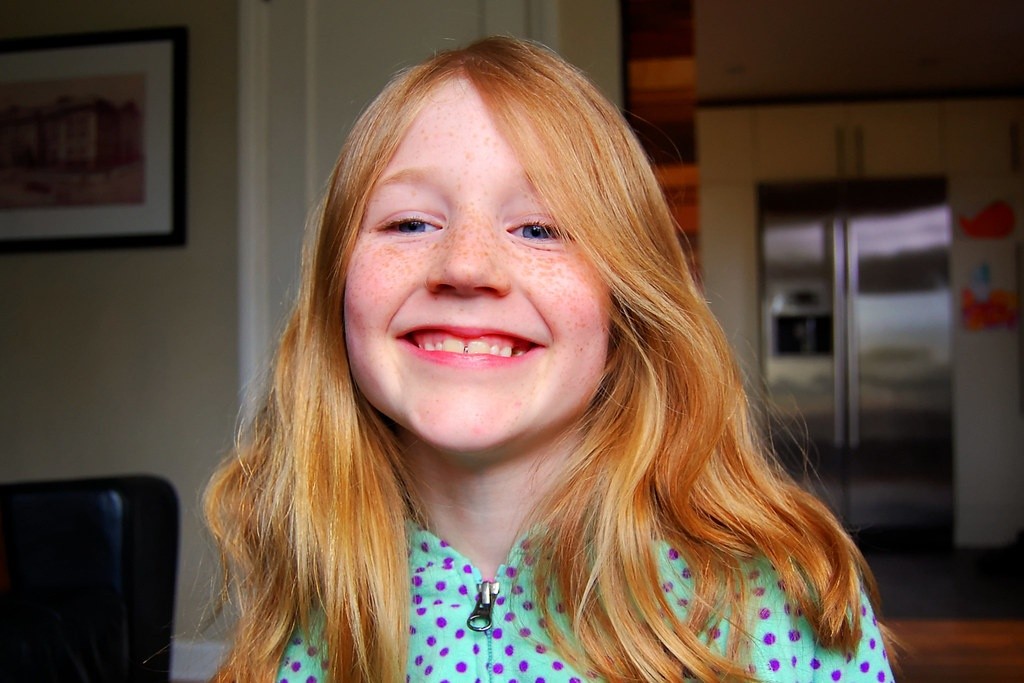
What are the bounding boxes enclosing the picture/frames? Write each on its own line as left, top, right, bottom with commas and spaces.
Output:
0, 31, 188, 251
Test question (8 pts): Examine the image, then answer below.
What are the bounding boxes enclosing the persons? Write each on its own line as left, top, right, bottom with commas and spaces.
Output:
201, 33, 900, 683
